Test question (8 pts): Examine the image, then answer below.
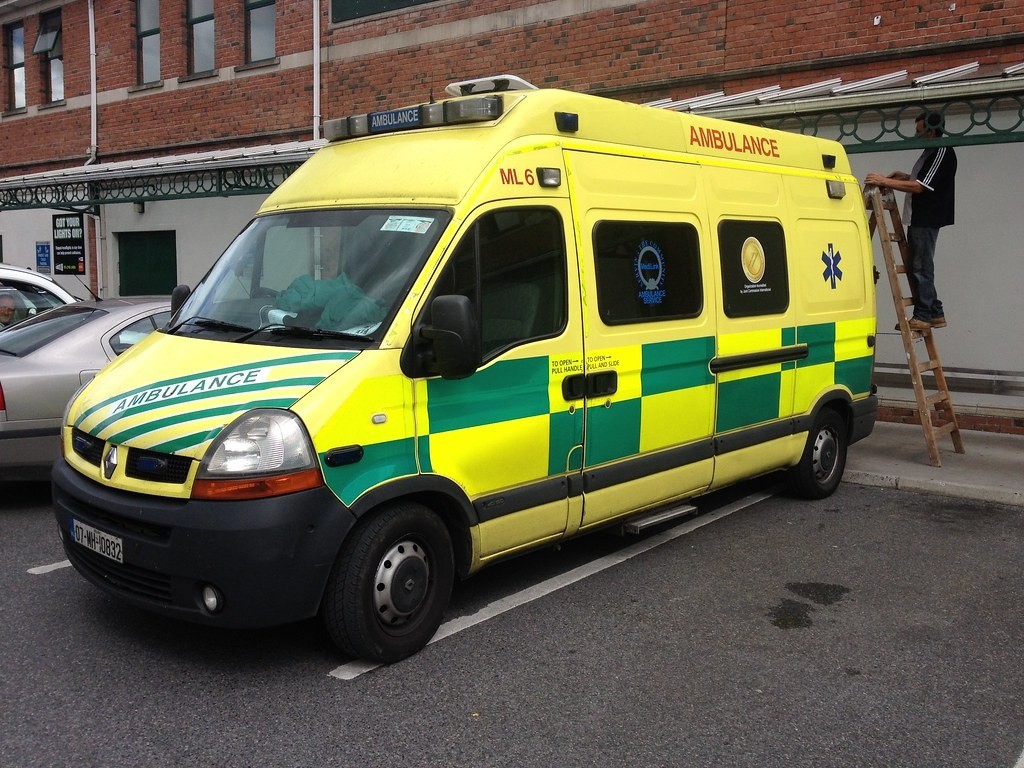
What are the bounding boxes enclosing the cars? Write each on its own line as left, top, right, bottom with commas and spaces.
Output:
0, 295, 223, 484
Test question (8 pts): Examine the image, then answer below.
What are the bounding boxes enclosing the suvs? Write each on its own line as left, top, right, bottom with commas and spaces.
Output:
0, 261, 152, 347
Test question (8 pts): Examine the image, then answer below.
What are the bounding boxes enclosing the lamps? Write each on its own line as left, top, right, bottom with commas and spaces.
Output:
133, 200, 144, 213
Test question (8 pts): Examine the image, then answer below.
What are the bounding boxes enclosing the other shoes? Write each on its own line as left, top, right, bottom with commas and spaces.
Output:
929, 316, 946, 328
895, 317, 931, 333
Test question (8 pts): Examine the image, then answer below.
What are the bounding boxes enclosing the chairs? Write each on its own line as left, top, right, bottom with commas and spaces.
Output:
472, 280, 549, 357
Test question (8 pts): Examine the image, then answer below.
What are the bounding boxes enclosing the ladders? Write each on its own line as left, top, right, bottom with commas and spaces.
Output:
862, 183, 967, 468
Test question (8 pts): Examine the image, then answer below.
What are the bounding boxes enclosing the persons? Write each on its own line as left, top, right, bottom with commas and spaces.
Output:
864, 111, 957, 333
0, 294, 19, 330
250, 247, 390, 331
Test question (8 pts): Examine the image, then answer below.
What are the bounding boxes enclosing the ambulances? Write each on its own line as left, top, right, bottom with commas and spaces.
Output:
49, 66, 881, 666
